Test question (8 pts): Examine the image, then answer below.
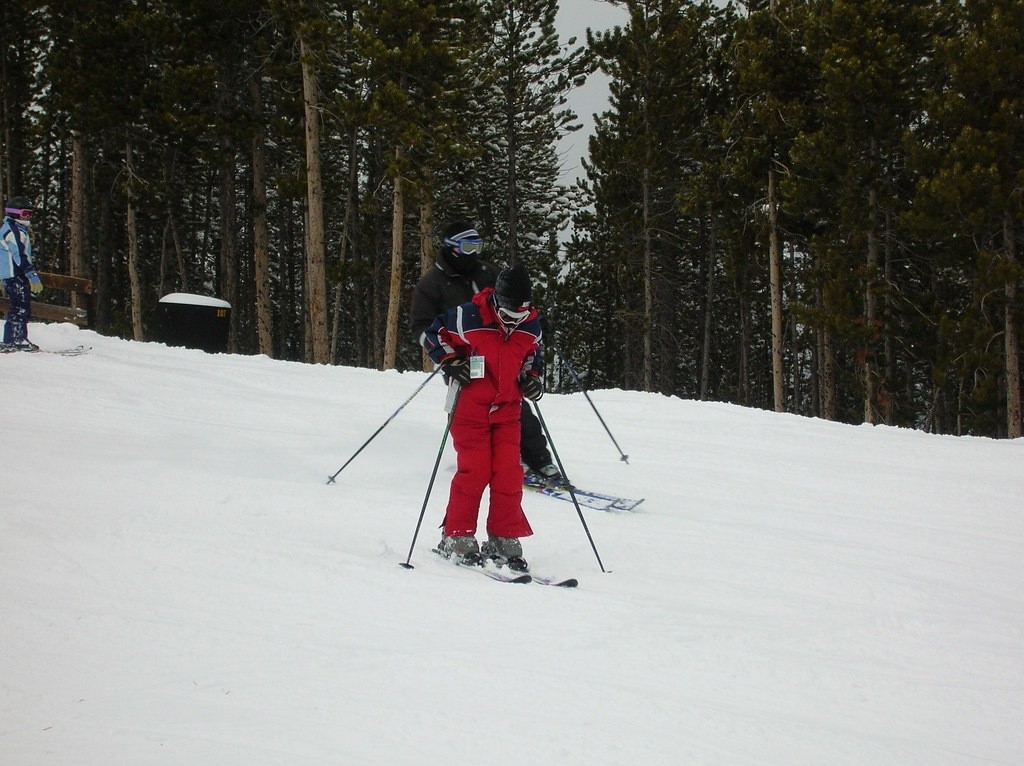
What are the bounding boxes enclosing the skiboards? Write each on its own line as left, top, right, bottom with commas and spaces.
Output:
430, 545, 581, 588
521, 469, 645, 511
0, 344, 94, 356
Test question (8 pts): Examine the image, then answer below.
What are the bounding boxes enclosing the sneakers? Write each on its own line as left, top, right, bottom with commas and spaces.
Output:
4, 337, 39, 352
441, 531, 483, 567
481, 534, 527, 571
520, 460, 536, 480
537, 463, 561, 479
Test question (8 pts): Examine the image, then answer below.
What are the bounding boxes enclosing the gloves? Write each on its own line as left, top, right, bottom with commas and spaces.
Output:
517, 373, 543, 401
24, 266, 43, 294
442, 359, 474, 386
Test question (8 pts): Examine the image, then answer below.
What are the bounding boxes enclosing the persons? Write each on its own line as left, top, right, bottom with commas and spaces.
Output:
419, 265, 578, 587
0, 196, 43, 353
409, 219, 646, 511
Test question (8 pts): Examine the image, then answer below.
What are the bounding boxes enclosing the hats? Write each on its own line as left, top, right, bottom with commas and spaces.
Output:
5, 197, 34, 219
495, 265, 531, 309
442, 220, 480, 248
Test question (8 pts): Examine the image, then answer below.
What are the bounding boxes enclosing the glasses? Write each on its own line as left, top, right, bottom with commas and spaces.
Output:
20, 208, 32, 219
498, 308, 530, 325
458, 239, 484, 255
